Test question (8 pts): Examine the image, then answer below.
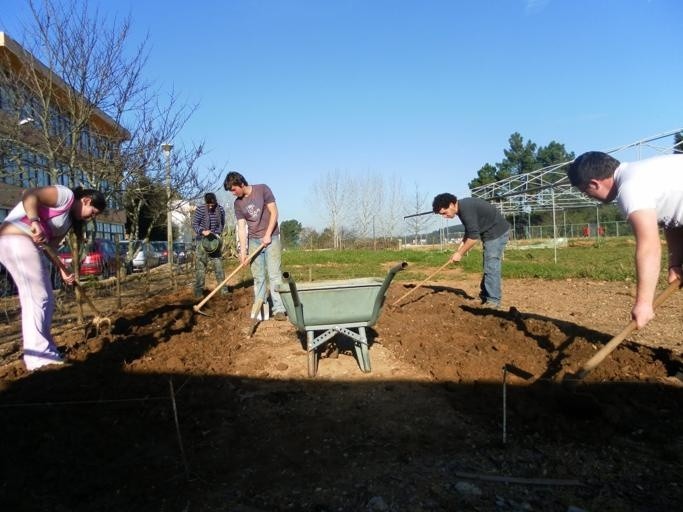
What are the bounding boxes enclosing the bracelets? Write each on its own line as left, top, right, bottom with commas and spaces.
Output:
28, 217, 40, 223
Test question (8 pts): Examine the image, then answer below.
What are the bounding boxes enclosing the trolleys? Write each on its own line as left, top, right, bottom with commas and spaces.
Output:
275, 262, 407, 377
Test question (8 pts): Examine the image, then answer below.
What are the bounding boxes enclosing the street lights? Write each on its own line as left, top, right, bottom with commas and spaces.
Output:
161, 143, 177, 281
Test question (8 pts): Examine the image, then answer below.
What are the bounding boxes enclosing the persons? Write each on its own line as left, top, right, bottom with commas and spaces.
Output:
191, 192, 233, 299
0, 183, 105, 370
222, 172, 286, 322
431, 192, 510, 309
567, 150, 683, 329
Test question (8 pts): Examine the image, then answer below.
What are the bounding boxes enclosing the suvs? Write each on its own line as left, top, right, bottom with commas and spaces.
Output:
62, 239, 130, 275
131, 240, 197, 270
1, 250, 61, 290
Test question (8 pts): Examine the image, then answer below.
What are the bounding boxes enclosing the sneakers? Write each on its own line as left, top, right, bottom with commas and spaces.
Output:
275, 312, 286, 320
474, 297, 497, 308
